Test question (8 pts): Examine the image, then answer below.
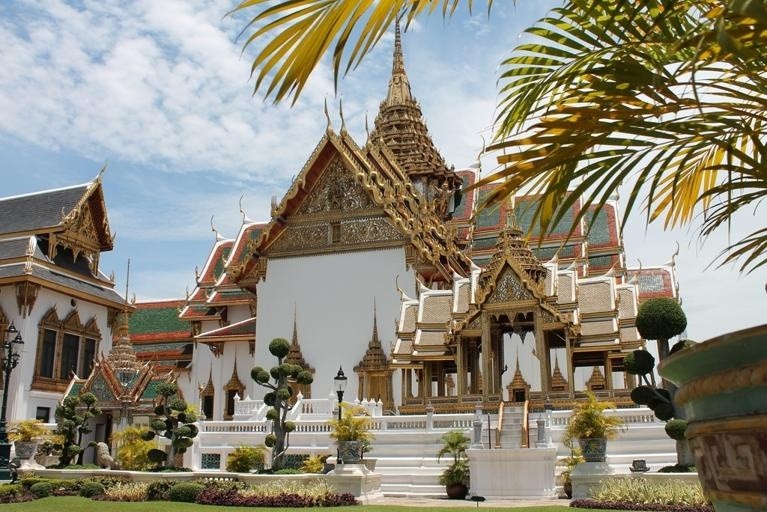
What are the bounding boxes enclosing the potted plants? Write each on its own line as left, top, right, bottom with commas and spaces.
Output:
325, 399, 379, 461
220, 0, 767, 512
438, 427, 472, 500
562, 382, 628, 462
5, 419, 50, 459
553, 456, 586, 498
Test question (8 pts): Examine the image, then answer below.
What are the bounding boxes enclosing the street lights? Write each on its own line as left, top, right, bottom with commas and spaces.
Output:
0, 319, 24, 440
332, 365, 347, 464
